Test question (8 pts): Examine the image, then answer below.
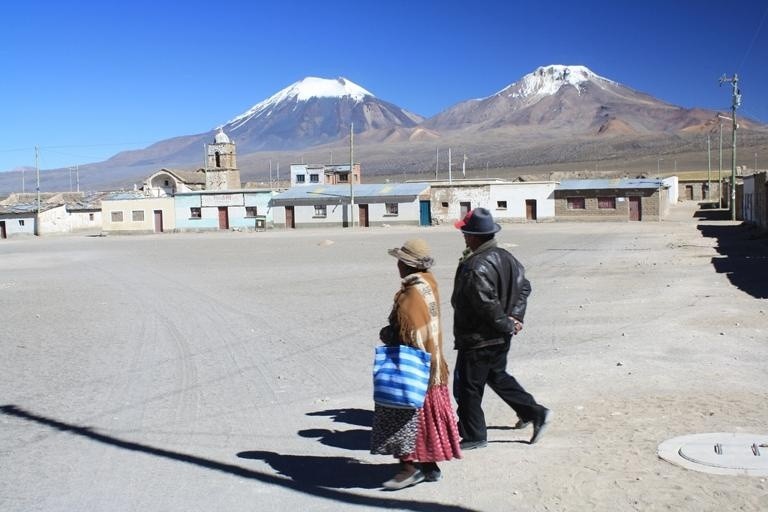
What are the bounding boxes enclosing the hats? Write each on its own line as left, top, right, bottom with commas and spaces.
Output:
453, 208, 502, 236
387, 238, 434, 271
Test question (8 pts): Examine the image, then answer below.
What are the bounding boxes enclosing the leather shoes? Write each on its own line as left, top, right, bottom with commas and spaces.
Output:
516, 408, 552, 445
458, 436, 497, 451
382, 466, 445, 490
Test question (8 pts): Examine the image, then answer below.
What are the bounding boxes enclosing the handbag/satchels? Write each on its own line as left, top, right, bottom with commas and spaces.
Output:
371, 343, 434, 409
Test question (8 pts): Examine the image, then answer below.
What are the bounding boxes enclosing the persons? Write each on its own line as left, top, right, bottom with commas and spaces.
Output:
455, 211, 534, 429
453, 208, 551, 449
371, 240, 459, 490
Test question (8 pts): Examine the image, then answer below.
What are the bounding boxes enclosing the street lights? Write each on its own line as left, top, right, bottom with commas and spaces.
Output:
718, 71, 743, 221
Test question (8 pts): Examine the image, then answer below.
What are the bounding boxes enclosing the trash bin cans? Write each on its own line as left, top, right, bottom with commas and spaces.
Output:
255, 215, 267, 233
